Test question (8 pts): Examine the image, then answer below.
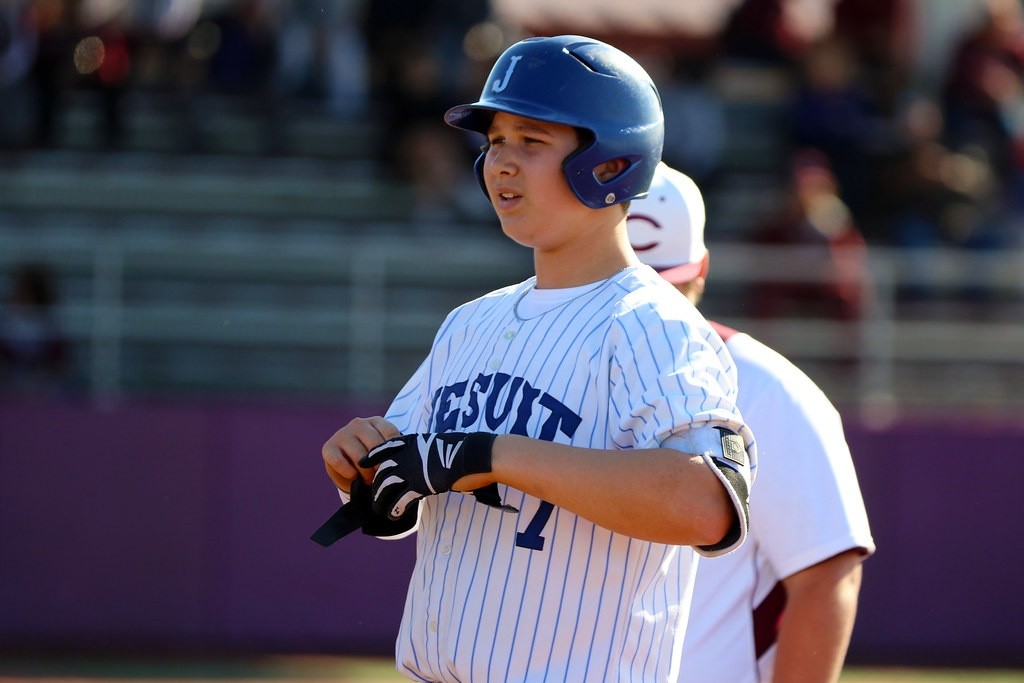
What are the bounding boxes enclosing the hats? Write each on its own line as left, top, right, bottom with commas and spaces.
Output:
444, 34, 664, 210
626, 162, 706, 286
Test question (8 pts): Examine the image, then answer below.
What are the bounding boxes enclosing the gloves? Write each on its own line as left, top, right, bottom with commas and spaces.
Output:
309, 432, 520, 548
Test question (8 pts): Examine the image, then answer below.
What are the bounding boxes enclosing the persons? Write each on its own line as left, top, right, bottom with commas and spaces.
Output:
2, 0, 1021, 321
317, 35, 759, 683
628, 163, 878, 682
